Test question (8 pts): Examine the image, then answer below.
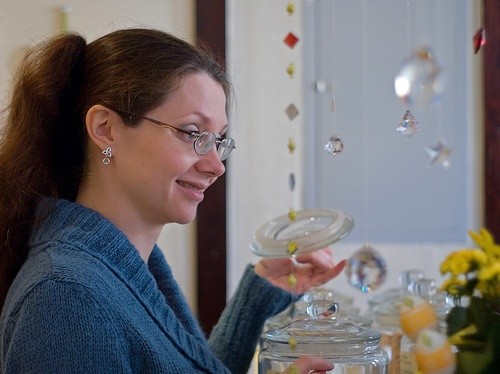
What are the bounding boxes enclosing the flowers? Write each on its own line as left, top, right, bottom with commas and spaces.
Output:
441, 227, 500, 374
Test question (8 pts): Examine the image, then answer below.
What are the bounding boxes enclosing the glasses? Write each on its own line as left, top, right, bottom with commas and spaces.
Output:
106, 105, 236, 161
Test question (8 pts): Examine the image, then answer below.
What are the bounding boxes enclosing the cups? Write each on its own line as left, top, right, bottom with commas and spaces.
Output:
401, 270, 455, 314
257, 288, 390, 374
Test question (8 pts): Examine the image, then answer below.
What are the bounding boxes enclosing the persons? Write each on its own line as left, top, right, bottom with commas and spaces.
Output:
0, 28, 349, 374
